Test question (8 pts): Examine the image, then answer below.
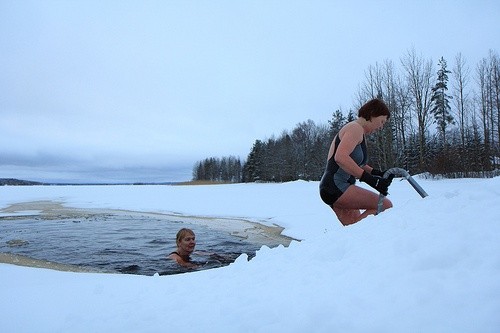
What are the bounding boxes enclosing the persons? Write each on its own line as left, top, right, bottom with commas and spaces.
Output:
320, 99, 393, 226
168, 228, 214, 269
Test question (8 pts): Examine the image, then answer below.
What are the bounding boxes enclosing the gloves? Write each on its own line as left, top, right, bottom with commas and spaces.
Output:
371, 168, 395, 182
359, 170, 391, 196
